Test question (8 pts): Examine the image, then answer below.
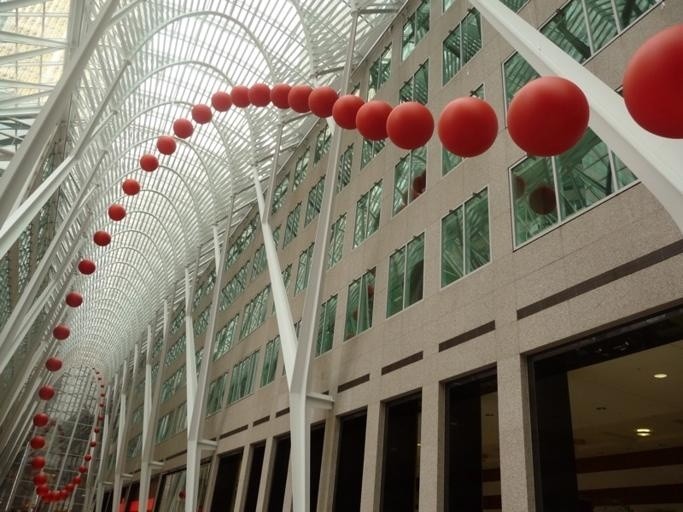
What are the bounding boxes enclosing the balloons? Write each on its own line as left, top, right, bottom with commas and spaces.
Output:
78, 179, 140, 274
437, 97, 498, 157
622, 26, 683, 139
507, 76, 590, 156
212, 83, 434, 150
29, 357, 105, 501
140, 104, 210, 172
52, 292, 82, 340
